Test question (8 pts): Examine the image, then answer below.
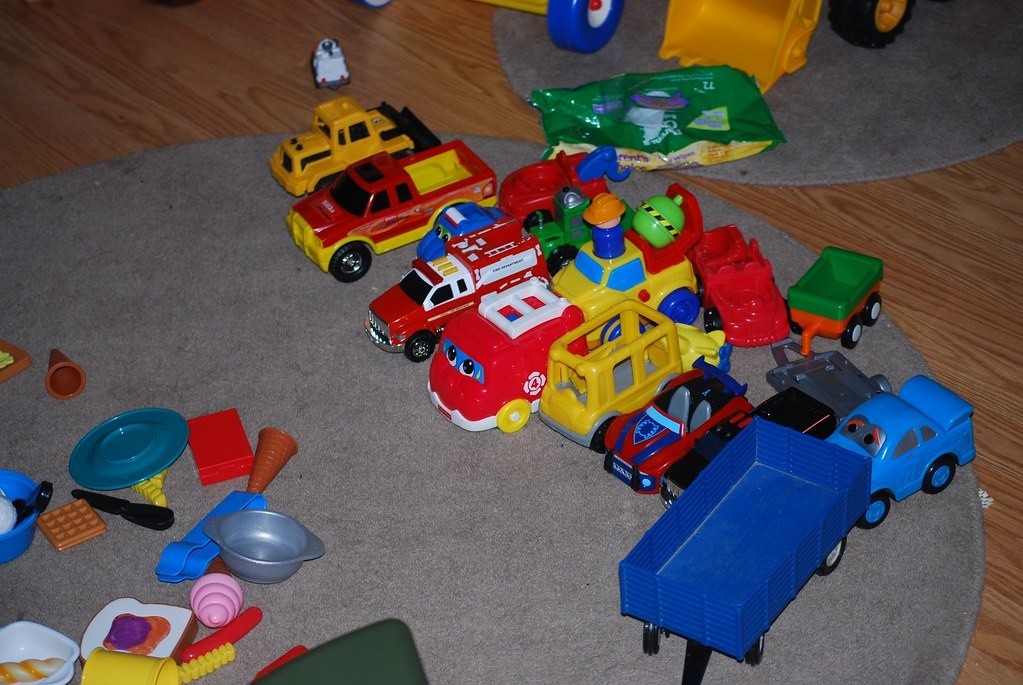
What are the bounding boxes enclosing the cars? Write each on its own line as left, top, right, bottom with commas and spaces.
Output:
270, 0, 979, 685
